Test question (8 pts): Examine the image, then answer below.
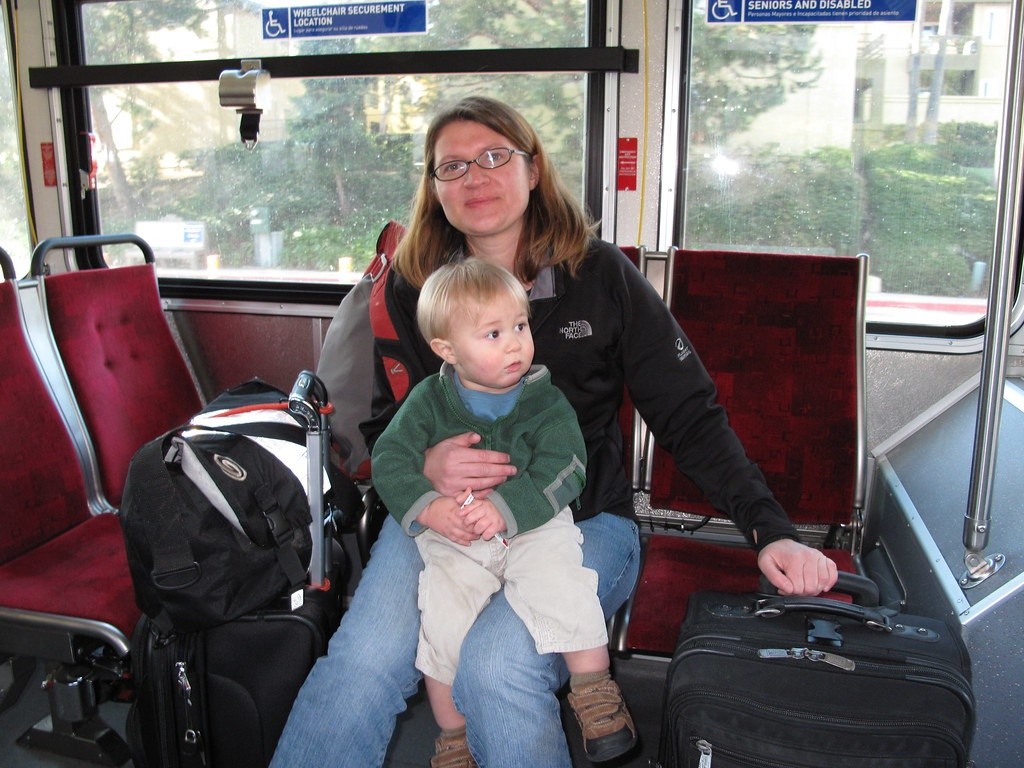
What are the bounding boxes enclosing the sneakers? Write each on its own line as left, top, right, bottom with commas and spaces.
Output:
569, 677, 637, 762
430, 724, 477, 768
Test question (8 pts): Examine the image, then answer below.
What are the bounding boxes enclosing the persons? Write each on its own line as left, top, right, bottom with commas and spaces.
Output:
267, 97, 837, 768
371, 255, 636, 768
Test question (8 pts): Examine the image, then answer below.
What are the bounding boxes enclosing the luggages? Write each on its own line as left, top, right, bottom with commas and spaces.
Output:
656, 569, 977, 768
120, 370, 348, 768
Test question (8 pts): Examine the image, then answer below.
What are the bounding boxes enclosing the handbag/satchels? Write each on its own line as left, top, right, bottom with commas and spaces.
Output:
118, 374, 342, 640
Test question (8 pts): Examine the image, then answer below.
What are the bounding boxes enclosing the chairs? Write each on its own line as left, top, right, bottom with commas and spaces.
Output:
611, 245, 869, 662
0, 249, 143, 768
29, 233, 209, 520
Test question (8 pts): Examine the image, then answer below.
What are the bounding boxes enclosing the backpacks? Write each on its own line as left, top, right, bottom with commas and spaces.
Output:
314, 220, 427, 485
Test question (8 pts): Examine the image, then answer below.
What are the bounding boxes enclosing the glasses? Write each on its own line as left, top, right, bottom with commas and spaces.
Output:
430, 146, 531, 181
